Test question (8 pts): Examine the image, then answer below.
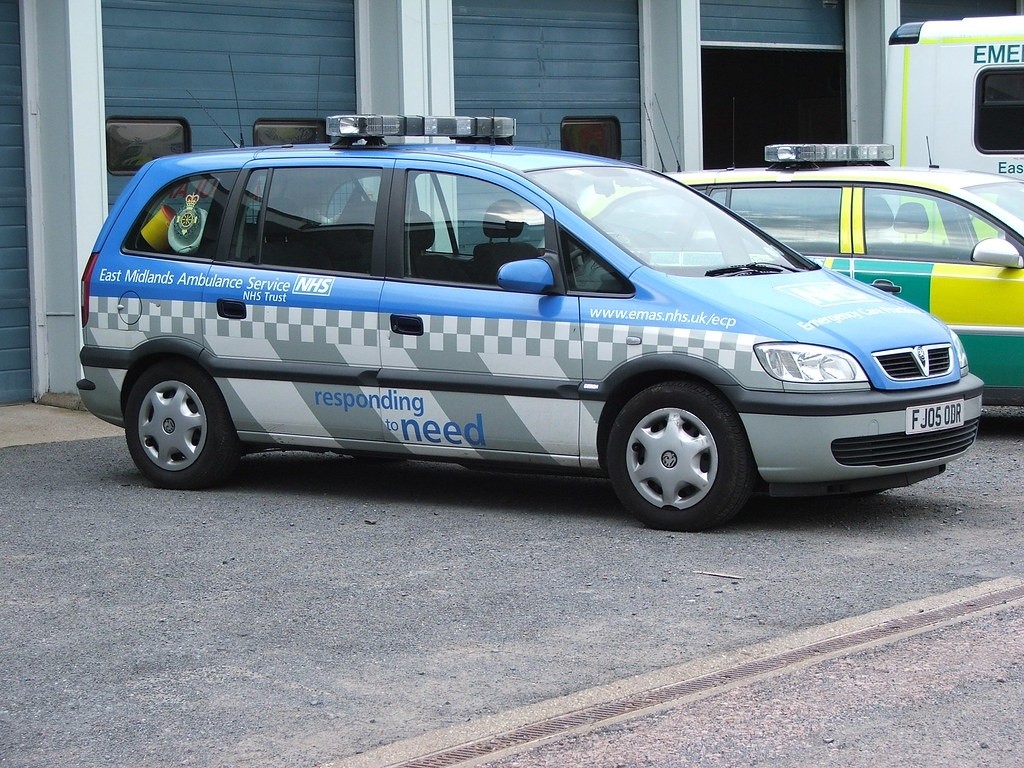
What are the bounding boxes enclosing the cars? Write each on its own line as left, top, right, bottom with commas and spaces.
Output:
70, 114, 984, 537
530, 139, 1023, 414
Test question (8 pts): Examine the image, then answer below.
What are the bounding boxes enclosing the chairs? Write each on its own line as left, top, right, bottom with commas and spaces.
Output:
895, 202, 937, 259
340, 201, 376, 249
407, 212, 471, 284
864, 196, 895, 257
473, 199, 539, 283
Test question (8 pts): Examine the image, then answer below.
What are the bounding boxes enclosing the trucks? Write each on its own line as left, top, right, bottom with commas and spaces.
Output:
880, 14, 1023, 245
